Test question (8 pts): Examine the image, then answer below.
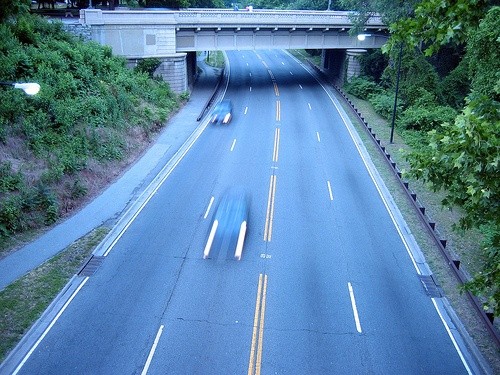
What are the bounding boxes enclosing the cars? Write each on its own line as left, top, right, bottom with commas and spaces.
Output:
209, 98, 233, 126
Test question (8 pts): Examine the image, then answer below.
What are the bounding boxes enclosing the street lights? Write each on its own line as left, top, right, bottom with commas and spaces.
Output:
357, 33, 404, 144
0, 82, 41, 95
